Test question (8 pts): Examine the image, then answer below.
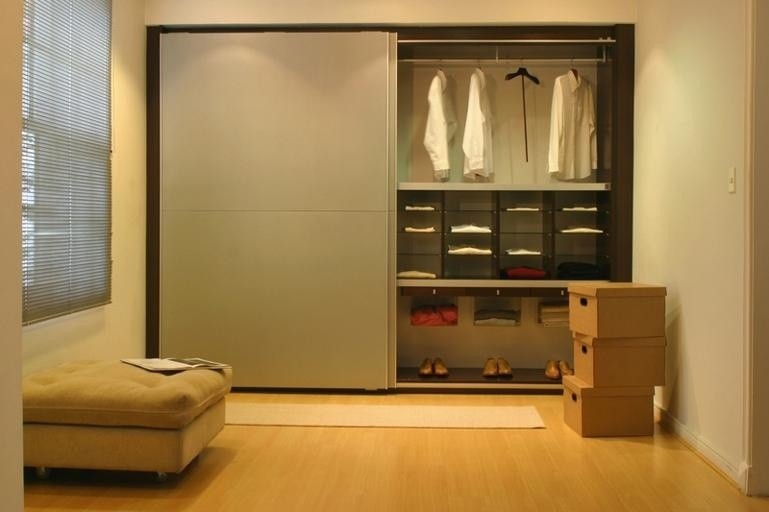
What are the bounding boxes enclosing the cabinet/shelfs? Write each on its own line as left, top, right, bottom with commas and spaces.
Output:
397, 190, 612, 279
146, 25, 633, 394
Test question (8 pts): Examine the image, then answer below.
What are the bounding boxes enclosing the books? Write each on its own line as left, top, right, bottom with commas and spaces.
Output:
119, 358, 233, 371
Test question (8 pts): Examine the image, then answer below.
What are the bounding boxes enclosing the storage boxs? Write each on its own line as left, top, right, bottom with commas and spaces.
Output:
564, 281, 669, 438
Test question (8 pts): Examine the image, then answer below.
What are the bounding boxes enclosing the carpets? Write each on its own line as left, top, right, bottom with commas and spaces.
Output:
224, 402, 547, 429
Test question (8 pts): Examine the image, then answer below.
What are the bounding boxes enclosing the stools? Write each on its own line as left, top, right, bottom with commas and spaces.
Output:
23, 358, 233, 483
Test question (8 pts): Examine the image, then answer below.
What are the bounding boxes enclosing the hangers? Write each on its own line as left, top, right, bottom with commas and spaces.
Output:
503, 57, 540, 87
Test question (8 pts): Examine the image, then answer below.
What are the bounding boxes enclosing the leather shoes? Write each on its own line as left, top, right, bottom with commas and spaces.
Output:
545, 360, 560, 379
418, 358, 433, 376
558, 360, 572, 376
432, 358, 449, 376
496, 357, 513, 376
480, 358, 498, 377
